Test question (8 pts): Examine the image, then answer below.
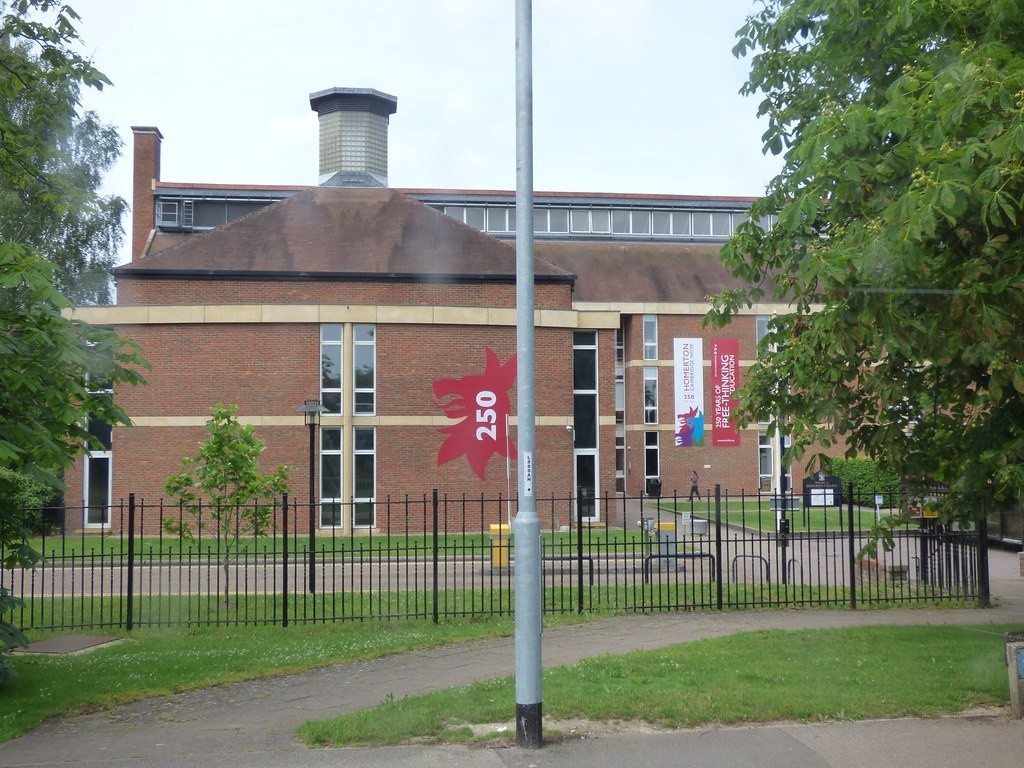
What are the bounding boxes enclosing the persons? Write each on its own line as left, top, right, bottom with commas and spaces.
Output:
687, 470, 702, 502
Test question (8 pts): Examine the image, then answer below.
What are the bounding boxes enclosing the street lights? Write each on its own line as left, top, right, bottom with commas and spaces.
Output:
296, 399, 331, 592
